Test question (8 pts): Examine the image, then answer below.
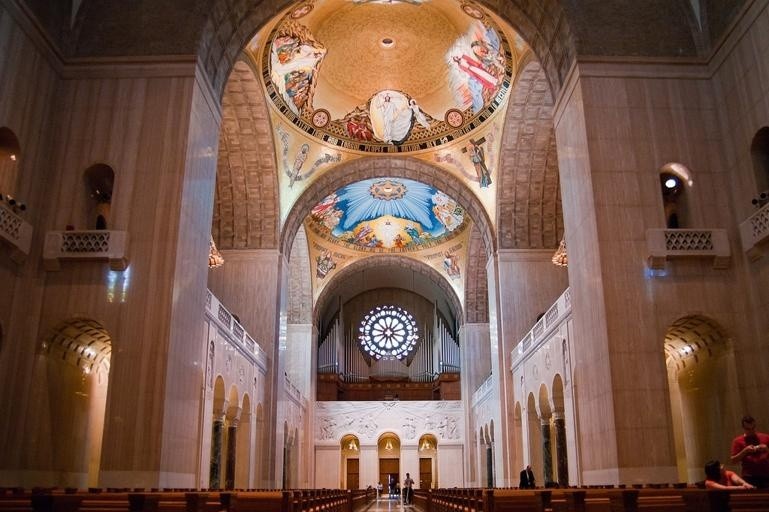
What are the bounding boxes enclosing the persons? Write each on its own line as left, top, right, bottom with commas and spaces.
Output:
402, 472, 415, 504
316, 248, 336, 280
728, 416, 769, 490
376, 481, 383, 496
388, 476, 396, 499
452, 53, 503, 93
705, 459, 754, 490
338, 94, 432, 145
274, 33, 327, 110
467, 143, 492, 188
288, 144, 309, 188
443, 250, 462, 282
519, 464, 536, 488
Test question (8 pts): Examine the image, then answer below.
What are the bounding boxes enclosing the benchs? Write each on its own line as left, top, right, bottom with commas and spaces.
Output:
411, 482, 768, 512
0, 486, 383, 511
